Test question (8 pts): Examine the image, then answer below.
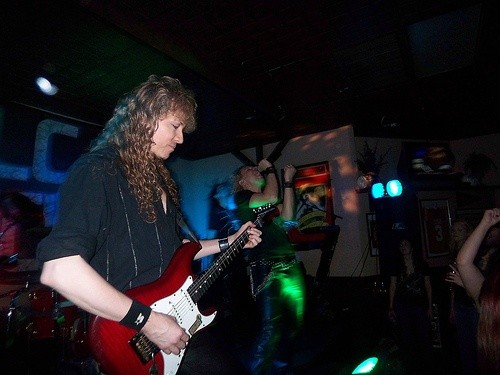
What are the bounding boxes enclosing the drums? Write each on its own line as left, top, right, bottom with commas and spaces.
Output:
9, 286, 76, 339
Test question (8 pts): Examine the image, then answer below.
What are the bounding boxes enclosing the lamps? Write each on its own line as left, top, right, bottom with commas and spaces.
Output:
386, 179, 403, 198
371, 182, 385, 199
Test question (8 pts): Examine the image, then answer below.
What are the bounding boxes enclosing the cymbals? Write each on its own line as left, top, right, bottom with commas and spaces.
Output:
27, 226, 55, 236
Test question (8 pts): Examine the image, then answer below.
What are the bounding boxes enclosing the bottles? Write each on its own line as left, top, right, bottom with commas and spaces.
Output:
447, 262, 457, 291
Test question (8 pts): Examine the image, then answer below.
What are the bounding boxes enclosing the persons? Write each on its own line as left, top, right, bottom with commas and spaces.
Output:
456, 207, 500, 375
485, 225, 499, 246
0, 198, 21, 298
388, 238, 432, 375
434, 219, 480, 375
444, 246, 498, 290
208, 183, 262, 304
232, 160, 306, 375
33, 74, 264, 375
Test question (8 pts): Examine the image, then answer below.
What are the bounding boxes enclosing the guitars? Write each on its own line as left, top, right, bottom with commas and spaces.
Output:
450, 257, 462, 329
85, 202, 280, 375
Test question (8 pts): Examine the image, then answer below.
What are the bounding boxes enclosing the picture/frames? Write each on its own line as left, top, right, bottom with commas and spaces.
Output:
366, 213, 379, 257
420, 198, 455, 259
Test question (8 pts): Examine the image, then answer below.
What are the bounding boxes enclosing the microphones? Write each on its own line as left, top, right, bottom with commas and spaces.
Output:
266, 150, 281, 163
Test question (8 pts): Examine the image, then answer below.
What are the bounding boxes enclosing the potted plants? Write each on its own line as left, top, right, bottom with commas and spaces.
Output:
354, 139, 393, 192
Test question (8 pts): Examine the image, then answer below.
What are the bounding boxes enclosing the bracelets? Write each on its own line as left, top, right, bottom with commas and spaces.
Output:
118, 300, 152, 331
388, 308, 393, 311
218, 238, 229, 252
284, 182, 294, 188
263, 167, 276, 176
